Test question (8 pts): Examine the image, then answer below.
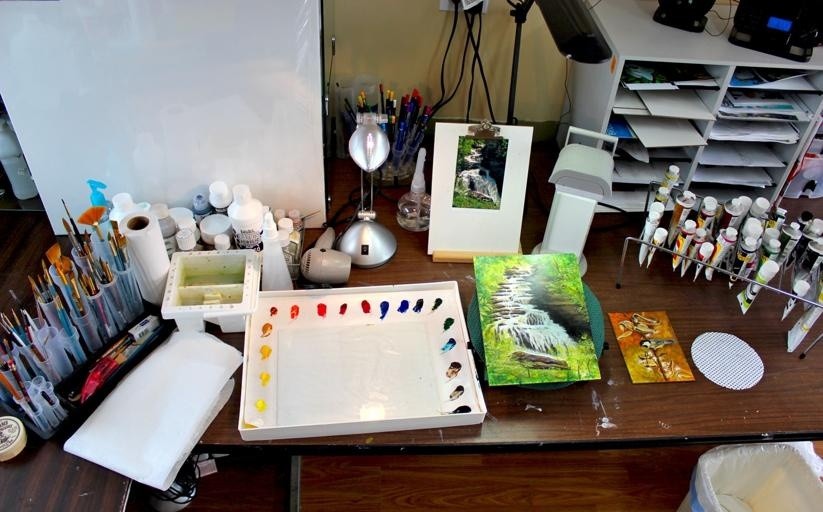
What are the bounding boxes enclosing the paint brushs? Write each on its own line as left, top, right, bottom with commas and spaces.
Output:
0, 199, 140, 382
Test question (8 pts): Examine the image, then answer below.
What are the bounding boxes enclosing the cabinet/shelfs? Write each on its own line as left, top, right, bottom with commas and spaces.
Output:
557, 0, 823, 213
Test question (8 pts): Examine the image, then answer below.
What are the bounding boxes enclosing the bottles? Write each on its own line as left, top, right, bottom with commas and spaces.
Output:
108, 181, 303, 249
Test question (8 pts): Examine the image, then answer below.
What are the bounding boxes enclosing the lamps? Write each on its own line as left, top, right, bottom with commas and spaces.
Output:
340, 113, 398, 268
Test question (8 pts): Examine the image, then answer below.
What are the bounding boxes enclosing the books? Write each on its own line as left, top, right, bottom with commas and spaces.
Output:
718, 67, 817, 122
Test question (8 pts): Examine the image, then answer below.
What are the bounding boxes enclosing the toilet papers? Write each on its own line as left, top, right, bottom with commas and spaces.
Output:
121, 212, 170, 304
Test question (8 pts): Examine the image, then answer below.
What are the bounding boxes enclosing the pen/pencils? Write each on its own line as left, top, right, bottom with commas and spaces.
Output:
343, 82, 432, 171
0, 340, 50, 430
79, 334, 139, 404
40, 392, 67, 423
